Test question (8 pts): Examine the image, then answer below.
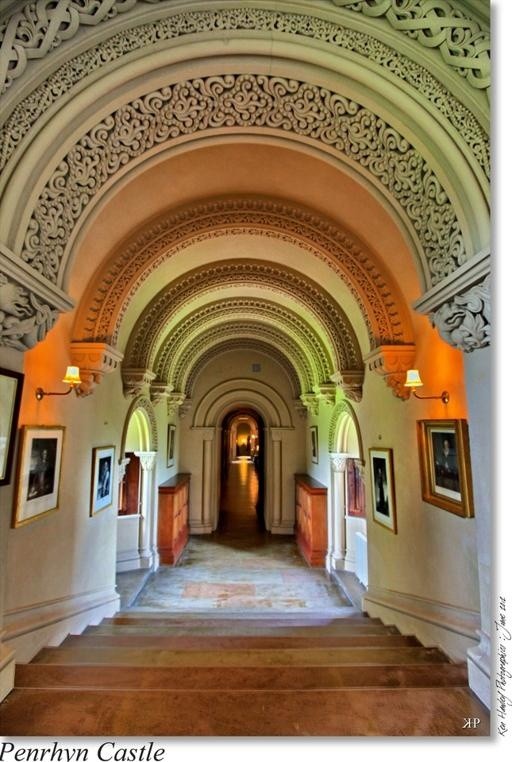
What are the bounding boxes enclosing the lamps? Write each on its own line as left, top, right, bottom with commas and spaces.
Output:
35, 366, 83, 404
402, 369, 450, 406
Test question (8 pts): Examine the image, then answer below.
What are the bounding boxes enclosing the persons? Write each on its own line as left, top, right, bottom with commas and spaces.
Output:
376, 466, 384, 510
441, 439, 453, 474
36, 448, 51, 492
101, 459, 109, 498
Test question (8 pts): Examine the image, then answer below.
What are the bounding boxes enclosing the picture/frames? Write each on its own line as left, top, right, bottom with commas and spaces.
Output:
0, 368, 25, 489
14, 423, 66, 524
167, 423, 177, 469
90, 446, 114, 518
309, 425, 319, 465
368, 446, 398, 533
416, 417, 474, 522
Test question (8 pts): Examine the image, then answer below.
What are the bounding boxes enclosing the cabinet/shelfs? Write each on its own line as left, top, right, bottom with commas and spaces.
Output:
294, 473, 328, 566
158, 471, 192, 566
